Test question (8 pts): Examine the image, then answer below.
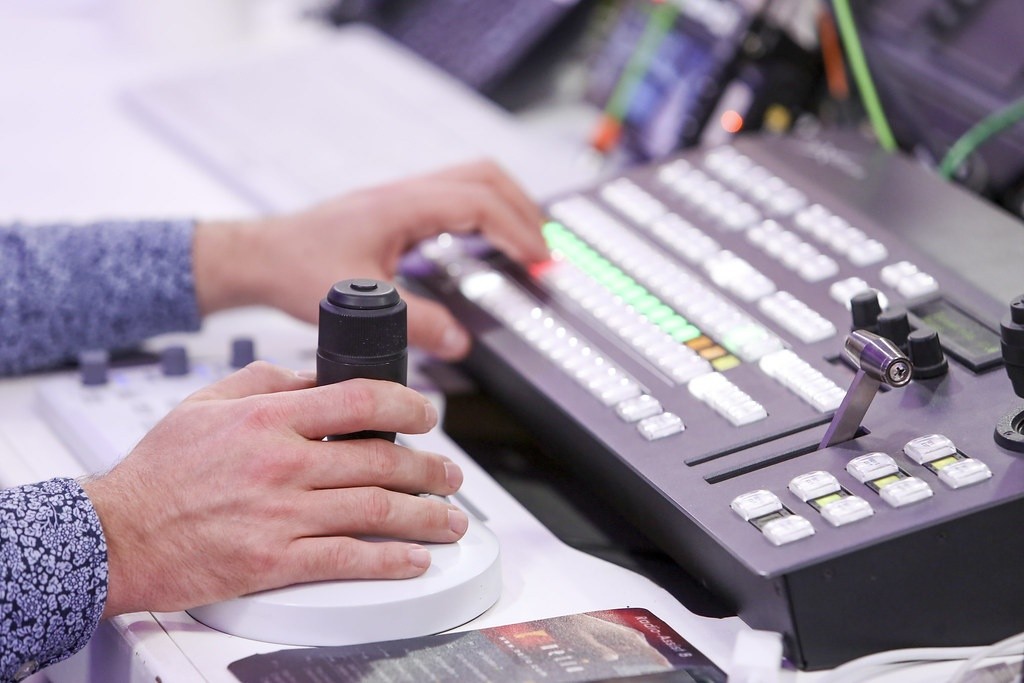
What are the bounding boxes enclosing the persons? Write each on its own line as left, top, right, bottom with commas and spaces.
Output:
0, 165, 545, 683
